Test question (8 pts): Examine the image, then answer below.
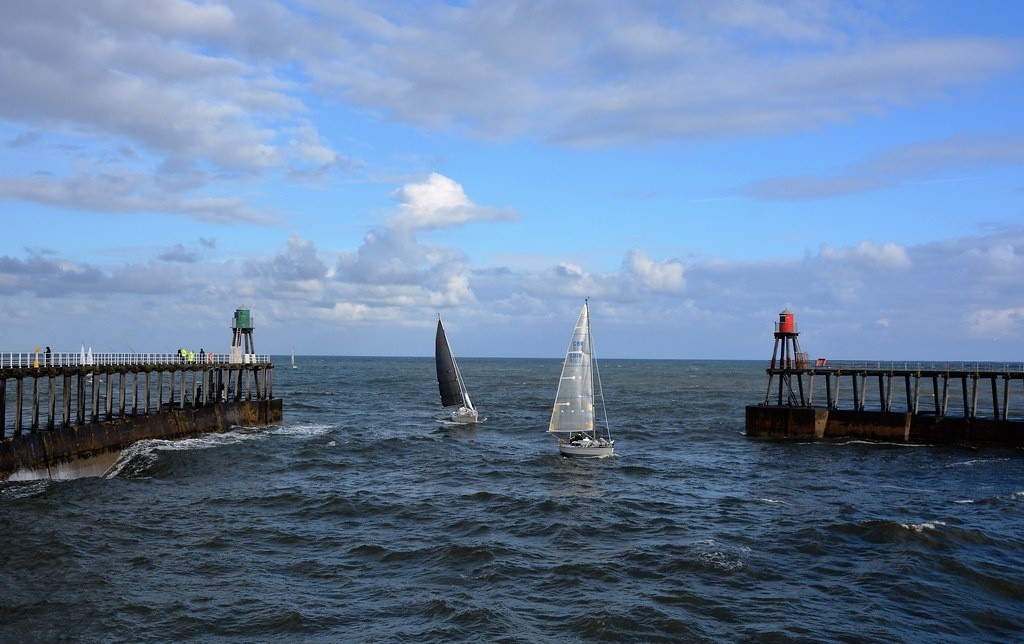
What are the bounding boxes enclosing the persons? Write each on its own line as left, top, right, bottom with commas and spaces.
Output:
177, 348, 196, 364
456, 404, 465, 411
200, 349, 205, 364
43, 347, 51, 366
570, 433, 583, 445
208, 353, 213, 363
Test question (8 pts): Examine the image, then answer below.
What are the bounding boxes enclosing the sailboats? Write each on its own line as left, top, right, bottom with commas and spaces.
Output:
436, 314, 479, 423
80, 345, 94, 379
545, 298, 614, 455
291, 348, 297, 369
